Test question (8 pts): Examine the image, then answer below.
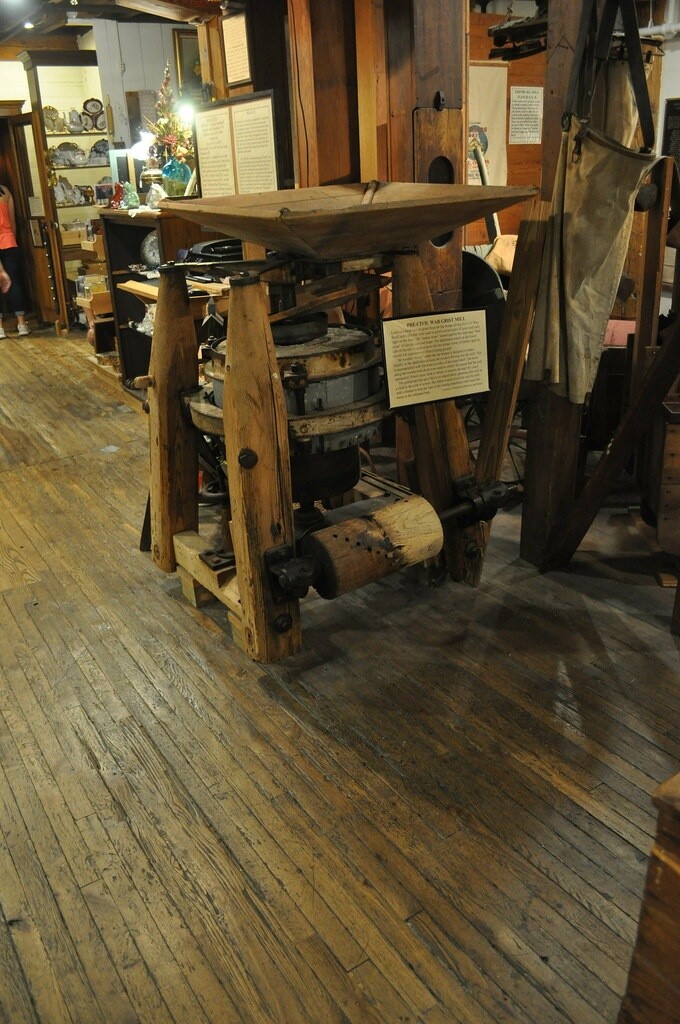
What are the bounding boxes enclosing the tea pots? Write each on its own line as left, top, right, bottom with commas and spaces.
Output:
67, 148, 92, 167
62, 107, 86, 135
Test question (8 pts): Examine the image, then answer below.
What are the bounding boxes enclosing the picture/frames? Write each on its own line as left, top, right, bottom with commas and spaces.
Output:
171, 28, 203, 102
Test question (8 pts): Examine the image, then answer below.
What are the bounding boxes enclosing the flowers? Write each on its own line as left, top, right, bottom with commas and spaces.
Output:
142, 56, 196, 163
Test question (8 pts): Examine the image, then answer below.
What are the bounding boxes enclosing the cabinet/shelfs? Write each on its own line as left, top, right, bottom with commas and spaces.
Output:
17, 50, 159, 404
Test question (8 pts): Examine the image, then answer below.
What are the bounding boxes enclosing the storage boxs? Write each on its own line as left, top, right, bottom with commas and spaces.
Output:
60, 228, 89, 246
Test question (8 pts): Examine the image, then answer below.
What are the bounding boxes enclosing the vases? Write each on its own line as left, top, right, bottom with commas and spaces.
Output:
140, 158, 162, 192
161, 156, 191, 196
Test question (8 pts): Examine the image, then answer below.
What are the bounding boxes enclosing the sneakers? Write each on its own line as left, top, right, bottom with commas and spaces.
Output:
0, 328, 6, 338
17, 322, 30, 335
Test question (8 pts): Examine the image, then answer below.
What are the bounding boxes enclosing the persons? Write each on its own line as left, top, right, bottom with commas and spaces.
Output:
0, 261, 11, 293
0, 184, 31, 338
